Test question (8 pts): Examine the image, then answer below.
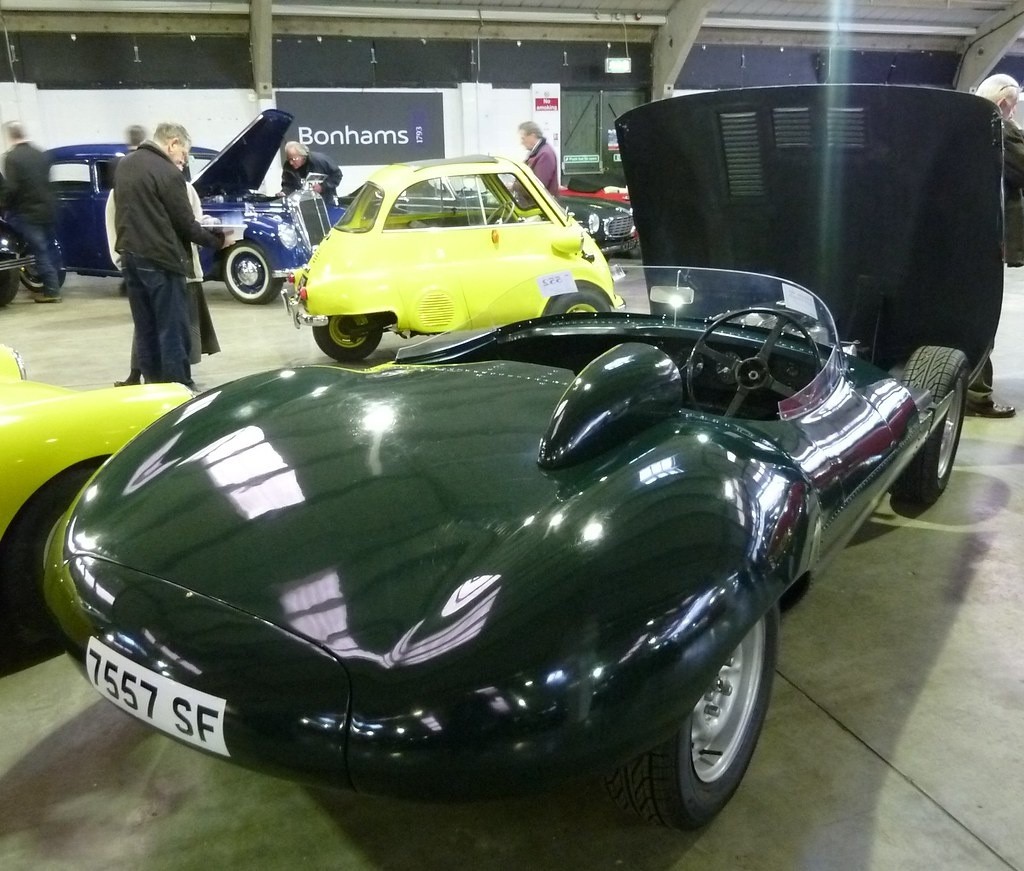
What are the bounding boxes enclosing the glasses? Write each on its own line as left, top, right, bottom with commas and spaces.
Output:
285, 157, 300, 160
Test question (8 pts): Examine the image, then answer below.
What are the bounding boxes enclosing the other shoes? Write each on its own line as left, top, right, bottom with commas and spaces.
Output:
114, 378, 141, 388
35, 295, 62, 303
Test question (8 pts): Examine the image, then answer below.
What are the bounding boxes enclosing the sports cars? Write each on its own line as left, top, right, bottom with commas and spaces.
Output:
45, 82, 1003, 835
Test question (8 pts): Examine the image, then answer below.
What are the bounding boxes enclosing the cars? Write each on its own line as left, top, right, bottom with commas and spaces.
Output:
303, 124, 637, 260
0, 340, 196, 658
0, 109, 313, 310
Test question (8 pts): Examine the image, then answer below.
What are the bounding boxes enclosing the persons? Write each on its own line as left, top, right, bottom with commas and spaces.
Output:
963, 74, 1024, 417
1, 120, 65, 304
505, 120, 558, 208
275, 141, 343, 206
105, 122, 224, 396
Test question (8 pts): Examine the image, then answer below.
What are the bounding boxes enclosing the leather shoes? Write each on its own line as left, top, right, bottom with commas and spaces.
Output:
964, 400, 1016, 419
